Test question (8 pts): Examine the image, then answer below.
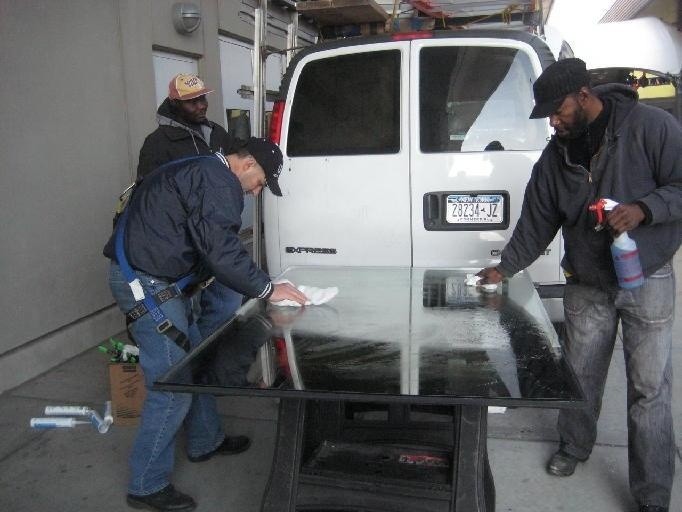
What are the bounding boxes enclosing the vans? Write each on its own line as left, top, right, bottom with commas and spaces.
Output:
259, 17, 681, 400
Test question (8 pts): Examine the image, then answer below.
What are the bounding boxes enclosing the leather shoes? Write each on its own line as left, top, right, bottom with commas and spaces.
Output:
546, 440, 593, 478
127, 482, 198, 512
187, 433, 251, 463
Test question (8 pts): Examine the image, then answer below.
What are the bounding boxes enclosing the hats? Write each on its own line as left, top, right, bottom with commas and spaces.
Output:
246, 137, 283, 196
529, 58, 587, 120
167, 73, 216, 101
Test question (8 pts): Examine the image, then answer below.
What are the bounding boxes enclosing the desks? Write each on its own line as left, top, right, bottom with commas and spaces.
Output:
148, 263, 586, 512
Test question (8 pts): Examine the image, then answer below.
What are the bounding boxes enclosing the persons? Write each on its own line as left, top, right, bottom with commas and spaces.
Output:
135, 72, 242, 342
94, 136, 310, 511
466, 56, 682, 512
